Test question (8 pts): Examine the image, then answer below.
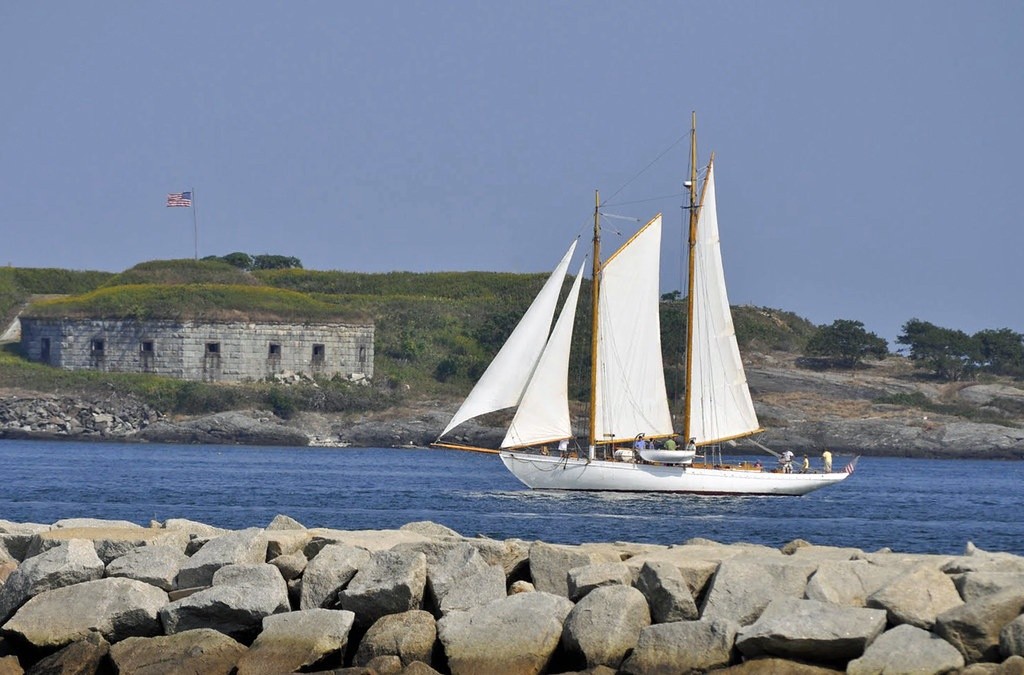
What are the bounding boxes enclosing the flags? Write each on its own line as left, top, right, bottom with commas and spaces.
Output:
166, 192, 191, 207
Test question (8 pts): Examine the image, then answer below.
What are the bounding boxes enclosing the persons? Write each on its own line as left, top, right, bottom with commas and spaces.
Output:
646, 435, 696, 467
801, 454, 809, 473
558, 438, 569, 457
821, 448, 833, 473
780, 448, 795, 473
634, 436, 645, 464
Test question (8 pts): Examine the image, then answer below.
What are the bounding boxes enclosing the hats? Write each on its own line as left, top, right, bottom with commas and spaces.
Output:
668, 435, 673, 439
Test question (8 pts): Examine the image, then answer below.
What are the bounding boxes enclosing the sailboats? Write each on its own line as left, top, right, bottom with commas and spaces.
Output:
430, 111, 862, 496
